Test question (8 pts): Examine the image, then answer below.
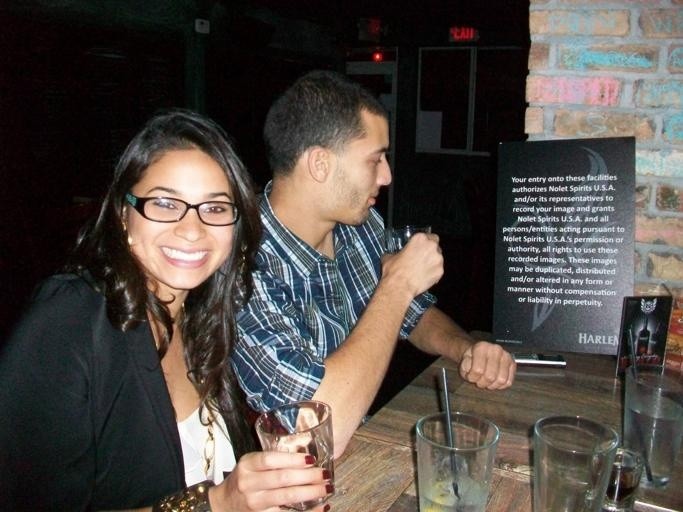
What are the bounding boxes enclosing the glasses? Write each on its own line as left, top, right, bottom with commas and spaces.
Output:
125, 191, 240, 226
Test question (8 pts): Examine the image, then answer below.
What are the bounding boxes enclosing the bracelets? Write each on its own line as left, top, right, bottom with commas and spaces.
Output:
153, 477, 217, 512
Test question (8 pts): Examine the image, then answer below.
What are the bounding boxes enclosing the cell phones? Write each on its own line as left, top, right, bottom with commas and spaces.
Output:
511, 352, 567, 368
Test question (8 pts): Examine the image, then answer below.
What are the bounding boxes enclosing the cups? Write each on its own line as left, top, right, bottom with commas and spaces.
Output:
255, 401, 336, 511
532, 364, 683, 512
386, 223, 431, 256
415, 411, 501, 511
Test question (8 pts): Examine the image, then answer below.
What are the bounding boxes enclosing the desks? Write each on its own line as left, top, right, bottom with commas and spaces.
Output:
324, 331, 683, 512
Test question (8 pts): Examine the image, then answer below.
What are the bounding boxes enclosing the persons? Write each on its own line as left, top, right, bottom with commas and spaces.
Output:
226, 62, 519, 453
0, 104, 337, 512
